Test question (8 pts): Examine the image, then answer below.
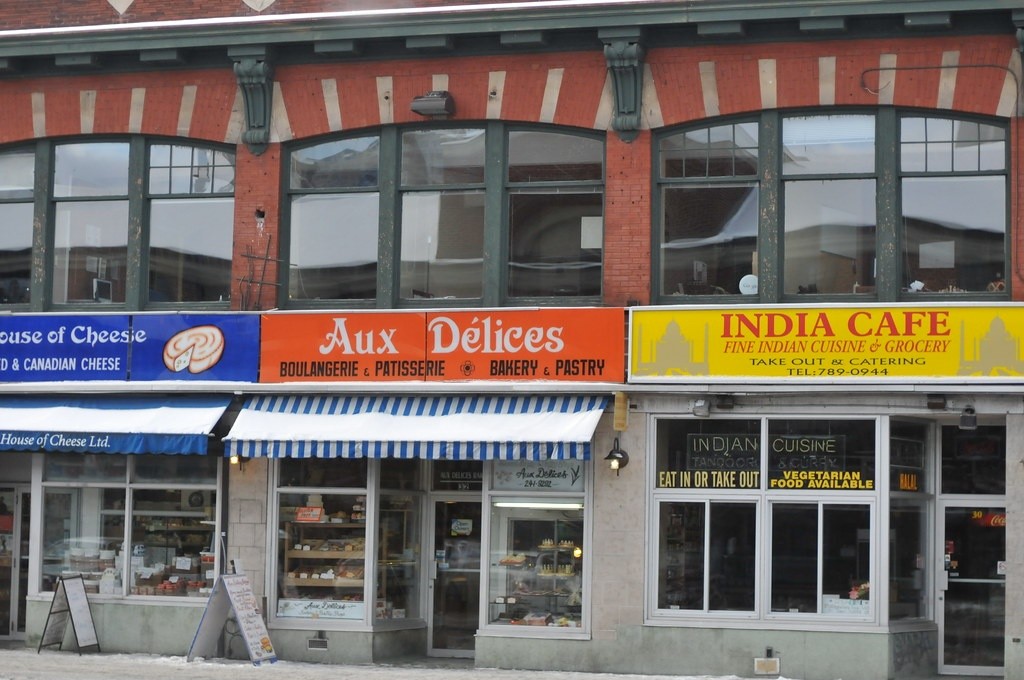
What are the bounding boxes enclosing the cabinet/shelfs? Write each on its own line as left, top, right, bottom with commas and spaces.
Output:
284, 519, 389, 601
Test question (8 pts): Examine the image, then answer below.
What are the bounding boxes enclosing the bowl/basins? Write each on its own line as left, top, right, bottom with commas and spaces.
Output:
199, 552, 214, 564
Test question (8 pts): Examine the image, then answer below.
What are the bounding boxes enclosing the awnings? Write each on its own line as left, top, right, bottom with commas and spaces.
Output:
222, 395, 610, 460
0, 394, 233, 456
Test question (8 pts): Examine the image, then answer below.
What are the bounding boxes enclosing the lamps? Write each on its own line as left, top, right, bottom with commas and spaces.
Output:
411, 91, 455, 116
693, 399, 711, 417
231, 455, 250, 471
604, 437, 629, 476
960, 405, 977, 429
